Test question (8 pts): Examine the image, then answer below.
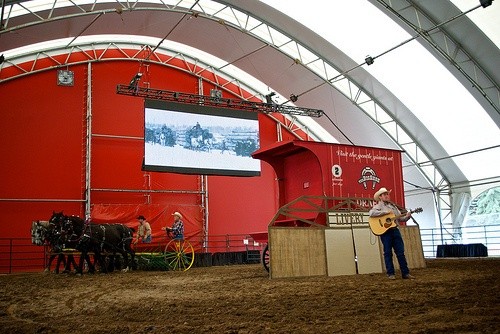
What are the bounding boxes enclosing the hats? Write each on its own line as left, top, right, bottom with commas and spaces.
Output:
373, 188, 392, 201
171, 212, 182, 219
136, 216, 146, 220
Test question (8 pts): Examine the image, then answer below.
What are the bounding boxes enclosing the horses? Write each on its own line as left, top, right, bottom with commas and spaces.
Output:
31, 211, 136, 274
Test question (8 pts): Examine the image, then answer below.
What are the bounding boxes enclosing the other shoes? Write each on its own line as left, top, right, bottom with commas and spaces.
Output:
406, 274, 416, 278
387, 274, 395, 279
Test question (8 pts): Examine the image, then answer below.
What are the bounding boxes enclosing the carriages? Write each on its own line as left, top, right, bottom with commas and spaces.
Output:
31, 209, 195, 277
159, 128, 213, 151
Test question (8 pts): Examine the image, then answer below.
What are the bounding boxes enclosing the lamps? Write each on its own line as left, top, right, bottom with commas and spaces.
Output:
478, 0, 495, 8
365, 56, 374, 65
131, 72, 144, 85
289, 93, 298, 103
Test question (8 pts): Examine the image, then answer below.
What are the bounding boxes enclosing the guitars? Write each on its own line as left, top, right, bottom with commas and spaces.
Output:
368, 207, 424, 236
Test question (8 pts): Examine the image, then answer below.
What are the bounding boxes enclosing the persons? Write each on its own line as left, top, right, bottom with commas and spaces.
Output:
153, 130, 158, 141
159, 128, 164, 135
369, 187, 415, 279
161, 212, 184, 241
195, 122, 200, 130
136, 216, 151, 242
